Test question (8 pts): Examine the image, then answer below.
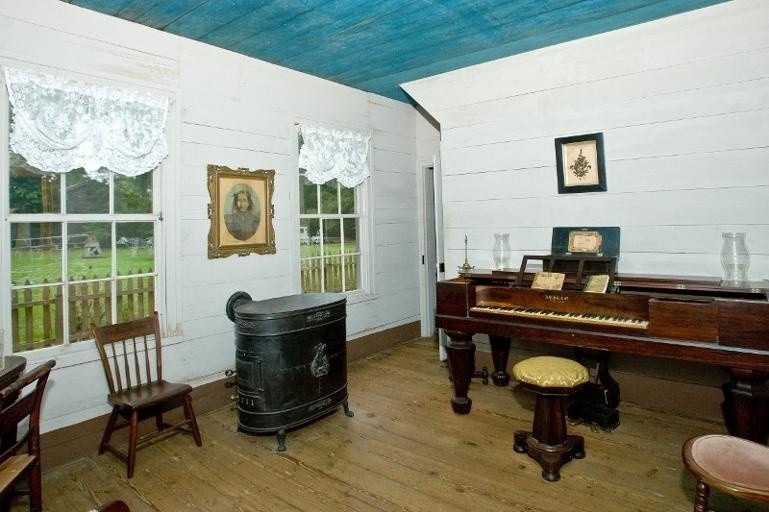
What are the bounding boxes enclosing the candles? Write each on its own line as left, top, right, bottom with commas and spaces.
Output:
458, 234, 475, 272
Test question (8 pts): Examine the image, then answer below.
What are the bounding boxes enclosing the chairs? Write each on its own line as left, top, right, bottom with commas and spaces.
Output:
1, 360, 56, 512
90, 311, 202, 478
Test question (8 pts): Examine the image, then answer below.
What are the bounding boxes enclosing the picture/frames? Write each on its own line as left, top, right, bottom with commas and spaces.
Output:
555, 132, 607, 194
207, 165, 275, 258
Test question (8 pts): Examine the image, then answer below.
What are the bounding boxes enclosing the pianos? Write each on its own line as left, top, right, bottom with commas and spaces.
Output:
434, 266, 764, 443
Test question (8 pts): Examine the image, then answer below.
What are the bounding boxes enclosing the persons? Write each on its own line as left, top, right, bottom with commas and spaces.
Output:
224, 190, 261, 241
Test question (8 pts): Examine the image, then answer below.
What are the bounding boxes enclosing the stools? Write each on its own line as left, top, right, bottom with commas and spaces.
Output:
512, 355, 589, 482
682, 432, 769, 512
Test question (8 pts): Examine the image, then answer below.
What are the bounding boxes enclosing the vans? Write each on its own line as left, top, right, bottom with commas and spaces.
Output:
300, 227, 326, 244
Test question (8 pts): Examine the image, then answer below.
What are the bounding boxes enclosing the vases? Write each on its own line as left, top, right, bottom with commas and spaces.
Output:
719, 231, 750, 288
493, 232, 511, 271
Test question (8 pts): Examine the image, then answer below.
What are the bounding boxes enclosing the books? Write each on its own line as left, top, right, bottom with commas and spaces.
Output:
531, 271, 566, 290
582, 274, 610, 293
